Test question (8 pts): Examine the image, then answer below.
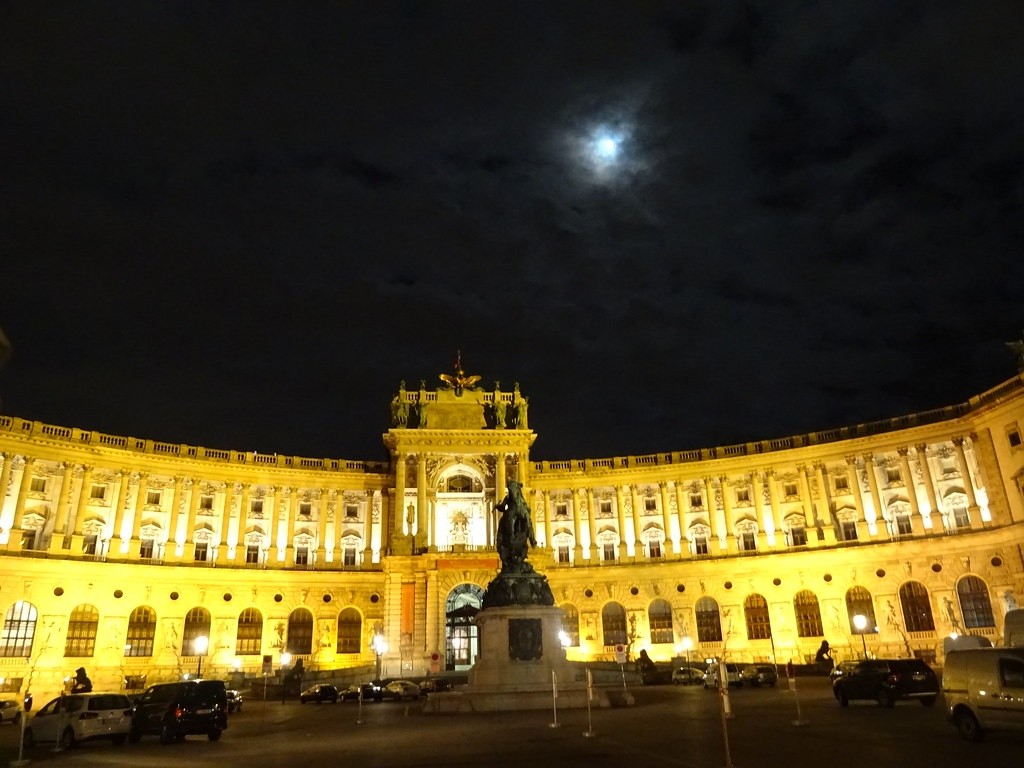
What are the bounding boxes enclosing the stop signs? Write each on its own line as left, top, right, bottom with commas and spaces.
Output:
615, 643, 627, 664
430, 650, 441, 664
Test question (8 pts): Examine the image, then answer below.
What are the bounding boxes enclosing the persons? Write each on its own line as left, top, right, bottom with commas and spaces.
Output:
493, 483, 538, 555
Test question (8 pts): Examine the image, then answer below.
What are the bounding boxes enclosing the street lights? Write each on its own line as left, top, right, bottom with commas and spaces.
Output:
192, 637, 208, 679
682, 639, 693, 686
853, 612, 868, 661
281, 653, 291, 705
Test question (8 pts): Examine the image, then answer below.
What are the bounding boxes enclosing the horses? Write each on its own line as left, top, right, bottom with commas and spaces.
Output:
490, 478, 538, 572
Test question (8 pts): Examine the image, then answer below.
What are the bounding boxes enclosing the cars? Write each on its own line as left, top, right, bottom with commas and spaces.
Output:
672, 663, 778, 689
944, 608, 1023, 740
830, 659, 940, 710
300, 677, 452, 707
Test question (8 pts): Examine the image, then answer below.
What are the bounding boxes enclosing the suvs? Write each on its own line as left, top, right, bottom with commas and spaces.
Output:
0, 700, 24, 724
22, 692, 129, 749
131, 678, 228, 745
